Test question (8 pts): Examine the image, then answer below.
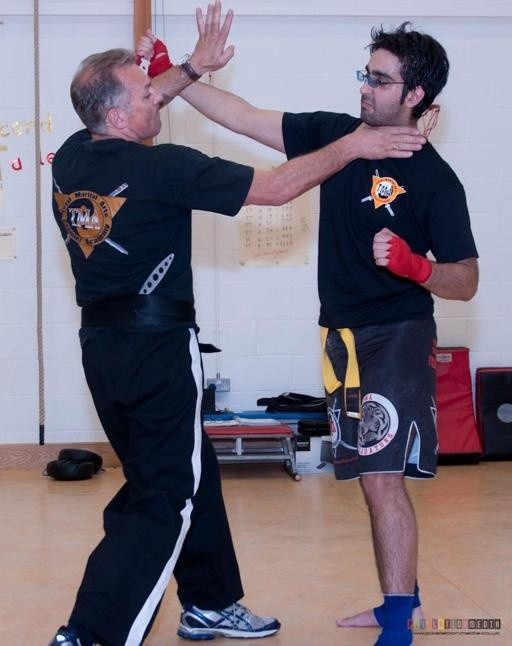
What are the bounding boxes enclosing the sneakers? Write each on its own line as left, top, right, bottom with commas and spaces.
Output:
173, 601, 282, 642
44, 624, 98, 646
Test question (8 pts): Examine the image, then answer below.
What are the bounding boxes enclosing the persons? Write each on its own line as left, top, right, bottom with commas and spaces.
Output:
50, 0, 431, 646
132, 20, 479, 645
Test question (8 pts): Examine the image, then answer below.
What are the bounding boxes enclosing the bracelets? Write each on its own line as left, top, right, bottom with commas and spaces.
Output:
181, 58, 205, 89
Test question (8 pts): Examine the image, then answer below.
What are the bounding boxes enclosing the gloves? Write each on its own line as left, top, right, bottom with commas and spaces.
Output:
382, 232, 435, 285
134, 38, 172, 79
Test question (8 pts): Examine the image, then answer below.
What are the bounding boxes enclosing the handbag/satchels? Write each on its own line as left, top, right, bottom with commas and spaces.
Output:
256, 390, 329, 413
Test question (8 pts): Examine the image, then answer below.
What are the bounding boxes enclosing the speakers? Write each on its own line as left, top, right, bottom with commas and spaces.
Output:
476, 367, 512, 461
